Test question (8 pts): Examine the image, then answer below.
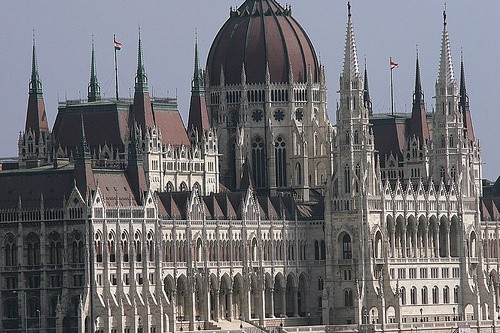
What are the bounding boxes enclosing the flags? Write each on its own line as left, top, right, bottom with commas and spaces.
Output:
113, 40, 123, 51
390, 60, 399, 70
